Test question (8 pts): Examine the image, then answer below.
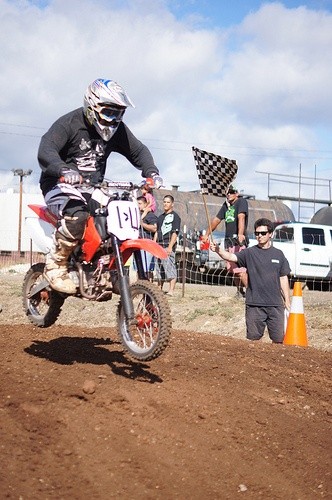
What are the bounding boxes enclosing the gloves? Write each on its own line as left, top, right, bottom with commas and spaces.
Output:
63, 169, 81, 185
150, 173, 163, 189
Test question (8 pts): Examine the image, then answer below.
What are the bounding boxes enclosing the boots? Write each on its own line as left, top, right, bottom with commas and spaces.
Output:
43, 231, 78, 294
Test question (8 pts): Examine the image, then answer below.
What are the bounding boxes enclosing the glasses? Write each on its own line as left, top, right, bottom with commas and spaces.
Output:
98, 106, 126, 122
227, 190, 238, 194
254, 231, 270, 236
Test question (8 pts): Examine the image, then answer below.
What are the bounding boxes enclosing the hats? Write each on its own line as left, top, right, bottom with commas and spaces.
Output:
229, 184, 238, 190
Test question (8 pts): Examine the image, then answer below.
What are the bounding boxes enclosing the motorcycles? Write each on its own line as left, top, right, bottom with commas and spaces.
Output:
20, 175, 172, 362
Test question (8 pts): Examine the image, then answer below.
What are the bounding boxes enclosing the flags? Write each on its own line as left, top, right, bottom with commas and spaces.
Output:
192, 146, 238, 197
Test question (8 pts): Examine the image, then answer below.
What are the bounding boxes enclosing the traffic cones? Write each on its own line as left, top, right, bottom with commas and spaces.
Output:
283, 281, 309, 347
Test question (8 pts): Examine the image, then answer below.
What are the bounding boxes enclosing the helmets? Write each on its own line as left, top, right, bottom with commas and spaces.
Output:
84, 78, 135, 141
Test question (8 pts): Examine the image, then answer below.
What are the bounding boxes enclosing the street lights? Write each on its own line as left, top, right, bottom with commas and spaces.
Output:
11, 167, 33, 255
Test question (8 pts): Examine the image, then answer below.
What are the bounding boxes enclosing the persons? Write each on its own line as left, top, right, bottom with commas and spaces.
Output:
202, 185, 250, 298
131, 196, 158, 283
140, 184, 156, 219
210, 217, 291, 345
37, 79, 163, 294
153, 194, 182, 296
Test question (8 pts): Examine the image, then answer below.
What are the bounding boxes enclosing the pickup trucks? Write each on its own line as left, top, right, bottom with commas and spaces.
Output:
206, 222, 332, 288
